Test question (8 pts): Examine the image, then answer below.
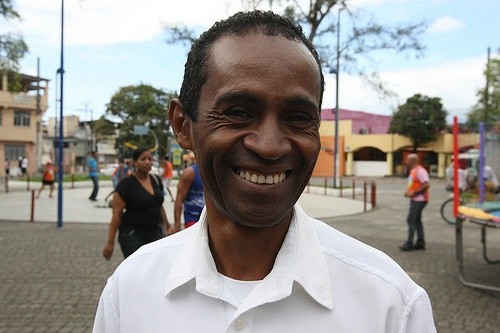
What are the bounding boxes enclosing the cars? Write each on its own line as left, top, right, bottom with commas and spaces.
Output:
444, 148, 480, 192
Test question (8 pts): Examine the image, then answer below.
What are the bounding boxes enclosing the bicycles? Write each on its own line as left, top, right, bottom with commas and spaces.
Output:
440, 174, 478, 224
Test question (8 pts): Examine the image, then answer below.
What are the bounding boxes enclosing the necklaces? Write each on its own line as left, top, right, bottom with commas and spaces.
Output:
160, 156, 176, 202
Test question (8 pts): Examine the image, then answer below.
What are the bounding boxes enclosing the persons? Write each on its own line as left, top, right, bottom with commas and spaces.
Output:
21, 156, 27, 175
102, 149, 170, 260
122, 160, 130, 179
91, 10, 436, 332
88, 150, 100, 200
171, 164, 204, 233
104, 158, 129, 202
473, 155, 500, 196
36, 160, 57, 199
4, 158, 10, 178
399, 154, 431, 250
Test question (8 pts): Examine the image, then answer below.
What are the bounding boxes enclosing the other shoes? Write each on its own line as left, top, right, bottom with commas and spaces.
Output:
413, 243, 425, 249
399, 241, 414, 250
